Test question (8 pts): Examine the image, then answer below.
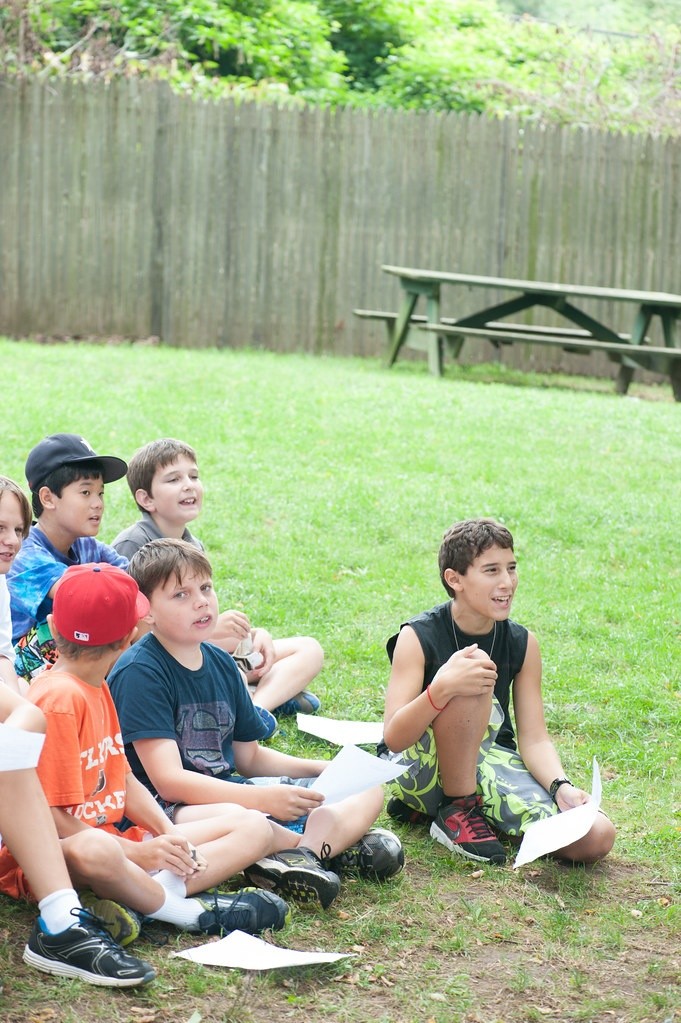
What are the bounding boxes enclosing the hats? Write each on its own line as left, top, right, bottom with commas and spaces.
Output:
25, 434, 128, 490
53, 562, 150, 646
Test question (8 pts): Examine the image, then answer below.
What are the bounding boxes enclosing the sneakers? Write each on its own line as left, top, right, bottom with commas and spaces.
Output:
250, 685, 320, 716
328, 828, 405, 880
23, 907, 157, 987
171, 886, 290, 938
430, 794, 507, 864
386, 796, 437, 826
77, 890, 143, 948
243, 842, 339, 911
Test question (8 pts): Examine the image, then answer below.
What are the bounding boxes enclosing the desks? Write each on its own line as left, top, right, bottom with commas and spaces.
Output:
381, 264, 681, 384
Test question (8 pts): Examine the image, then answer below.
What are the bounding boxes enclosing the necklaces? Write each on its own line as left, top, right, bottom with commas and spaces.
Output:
450, 602, 496, 658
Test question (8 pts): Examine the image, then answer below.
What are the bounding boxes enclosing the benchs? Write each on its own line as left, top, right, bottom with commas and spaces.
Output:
352, 305, 651, 385
410, 322, 681, 404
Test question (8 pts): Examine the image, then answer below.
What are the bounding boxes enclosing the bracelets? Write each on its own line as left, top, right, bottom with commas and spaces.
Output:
427, 684, 444, 711
550, 777, 575, 805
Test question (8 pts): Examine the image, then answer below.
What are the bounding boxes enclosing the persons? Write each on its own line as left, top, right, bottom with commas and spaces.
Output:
105, 537, 405, 914
0, 678, 158, 989
0, 474, 33, 697
22, 561, 288, 947
7, 434, 138, 683
376, 517, 616, 867
110, 437, 323, 740
